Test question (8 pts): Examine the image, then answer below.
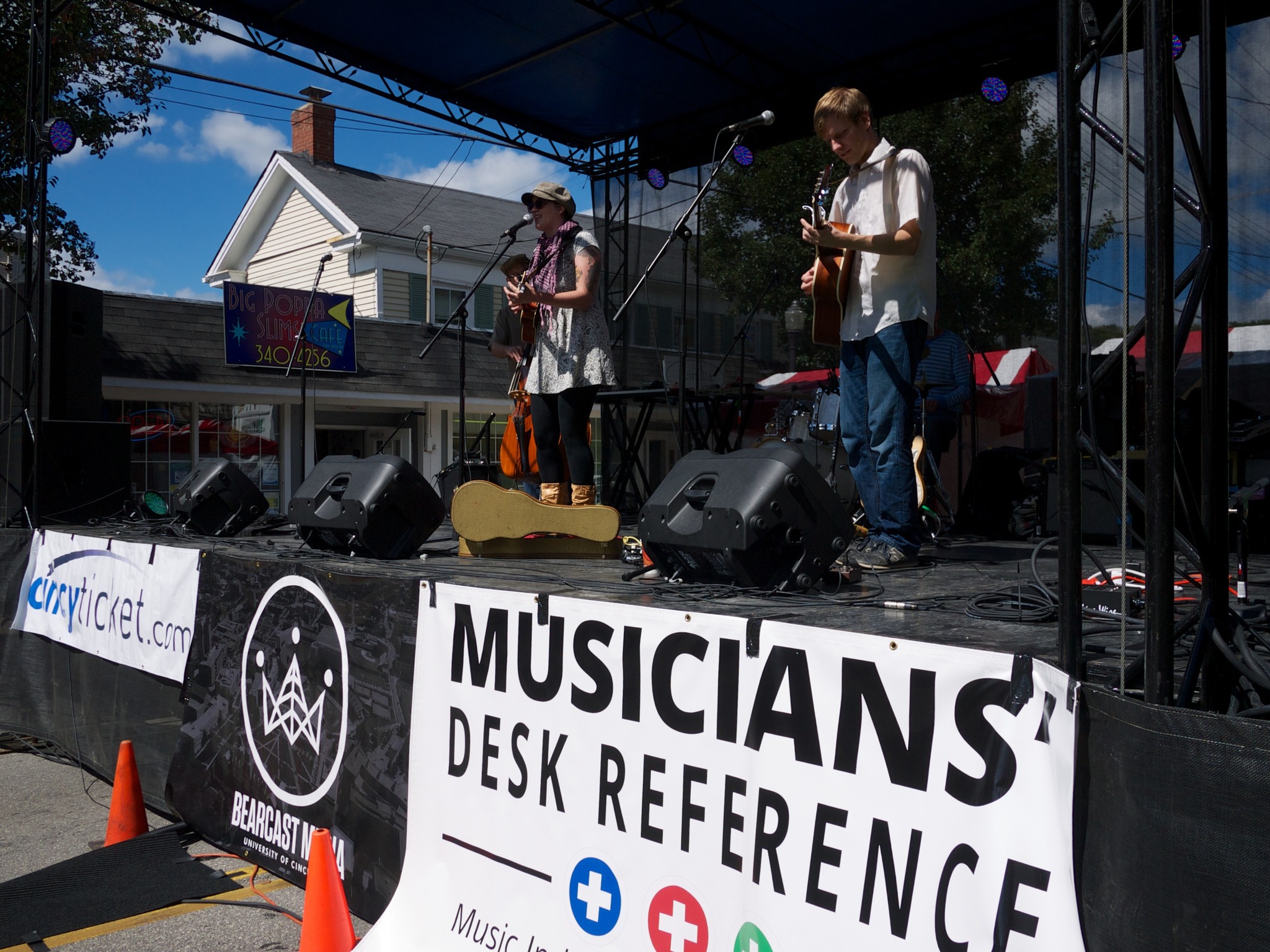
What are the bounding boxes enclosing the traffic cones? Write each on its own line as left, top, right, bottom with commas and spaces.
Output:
296, 826, 362, 952
101, 740, 150, 849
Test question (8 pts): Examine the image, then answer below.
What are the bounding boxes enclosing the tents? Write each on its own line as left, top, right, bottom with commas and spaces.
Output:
126, 422, 281, 458
1088, 323, 1270, 369
752, 347, 1057, 439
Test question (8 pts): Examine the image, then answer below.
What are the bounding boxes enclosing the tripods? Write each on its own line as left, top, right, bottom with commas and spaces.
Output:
605, 129, 745, 584
249, 260, 327, 539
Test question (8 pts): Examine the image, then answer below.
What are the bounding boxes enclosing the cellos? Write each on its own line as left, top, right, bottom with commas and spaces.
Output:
498, 342, 590, 483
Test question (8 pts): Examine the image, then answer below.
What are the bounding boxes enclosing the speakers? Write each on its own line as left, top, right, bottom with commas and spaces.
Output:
0, 279, 106, 423
1046, 467, 1126, 537
973, 444, 1044, 544
438, 463, 498, 516
1023, 376, 1057, 460
1, 413, 133, 529
1079, 352, 1138, 453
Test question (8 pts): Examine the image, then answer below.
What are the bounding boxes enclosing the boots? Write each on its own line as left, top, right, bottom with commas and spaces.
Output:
541, 482, 570, 504
571, 485, 597, 505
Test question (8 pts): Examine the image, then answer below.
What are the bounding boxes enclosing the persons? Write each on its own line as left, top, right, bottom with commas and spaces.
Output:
503, 180, 617, 541
487, 253, 576, 508
798, 87, 939, 572
907, 300, 978, 513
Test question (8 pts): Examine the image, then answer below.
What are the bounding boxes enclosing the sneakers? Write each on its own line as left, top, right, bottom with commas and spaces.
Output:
836, 539, 918, 569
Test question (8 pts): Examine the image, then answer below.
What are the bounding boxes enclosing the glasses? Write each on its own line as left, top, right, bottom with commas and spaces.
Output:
527, 198, 550, 213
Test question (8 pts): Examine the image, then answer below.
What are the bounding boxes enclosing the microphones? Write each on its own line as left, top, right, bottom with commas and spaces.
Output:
407, 411, 428, 416
320, 252, 333, 263
499, 213, 534, 238
721, 109, 777, 135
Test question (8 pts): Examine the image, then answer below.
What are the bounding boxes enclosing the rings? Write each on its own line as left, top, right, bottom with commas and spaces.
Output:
512, 298, 516, 302
809, 239, 813, 244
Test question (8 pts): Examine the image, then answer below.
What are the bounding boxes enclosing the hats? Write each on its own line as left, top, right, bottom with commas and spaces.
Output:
500, 254, 530, 274
521, 181, 577, 220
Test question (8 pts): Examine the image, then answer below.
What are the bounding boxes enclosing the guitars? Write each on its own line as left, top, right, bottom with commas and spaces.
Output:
516, 270, 541, 344
857, 433, 926, 513
804, 163, 855, 351
